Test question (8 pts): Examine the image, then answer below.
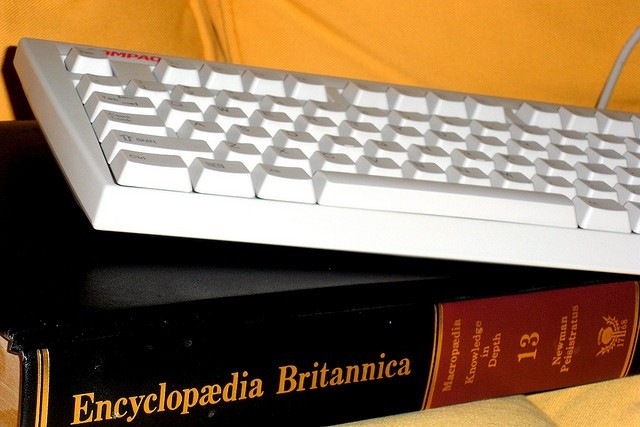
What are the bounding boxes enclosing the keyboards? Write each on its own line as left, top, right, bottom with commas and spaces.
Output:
12, 38, 640, 277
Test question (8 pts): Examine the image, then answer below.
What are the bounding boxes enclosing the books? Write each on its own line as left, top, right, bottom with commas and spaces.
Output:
0, 117, 640, 427
